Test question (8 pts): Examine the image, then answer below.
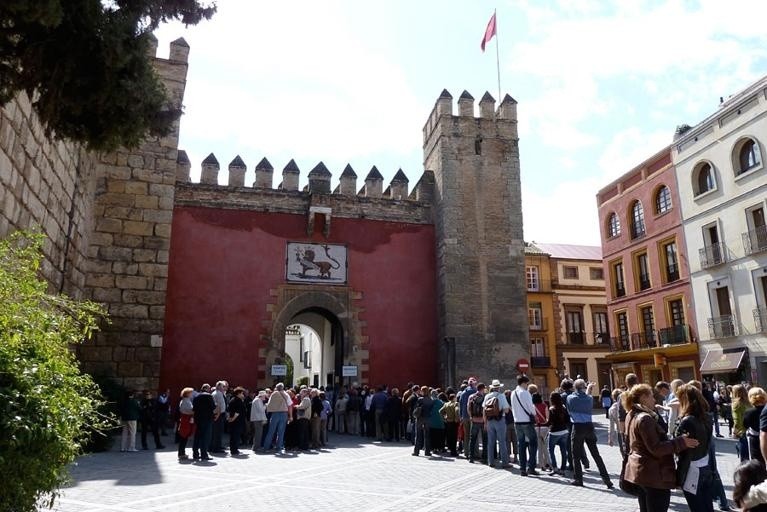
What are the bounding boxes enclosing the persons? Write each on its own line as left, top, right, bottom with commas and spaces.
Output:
335, 375, 613, 487
123, 374, 333, 462
599, 374, 766, 511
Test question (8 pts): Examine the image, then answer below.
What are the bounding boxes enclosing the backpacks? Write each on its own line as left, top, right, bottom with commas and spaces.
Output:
484, 392, 499, 416
469, 394, 483, 416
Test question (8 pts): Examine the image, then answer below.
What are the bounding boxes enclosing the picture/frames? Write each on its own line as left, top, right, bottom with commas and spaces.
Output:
284, 240, 349, 286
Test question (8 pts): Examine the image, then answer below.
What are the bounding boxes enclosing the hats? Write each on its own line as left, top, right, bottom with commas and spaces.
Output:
257, 390, 267, 396
489, 379, 503, 388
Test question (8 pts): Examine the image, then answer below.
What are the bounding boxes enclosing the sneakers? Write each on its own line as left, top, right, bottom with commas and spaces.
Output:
520, 471, 528, 476
528, 469, 538, 475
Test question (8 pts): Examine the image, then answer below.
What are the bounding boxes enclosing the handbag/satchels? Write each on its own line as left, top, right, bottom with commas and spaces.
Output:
530, 414, 535, 423
619, 453, 643, 496
412, 407, 421, 419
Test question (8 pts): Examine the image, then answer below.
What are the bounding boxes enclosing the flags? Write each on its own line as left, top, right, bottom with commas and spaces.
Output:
481, 12, 496, 52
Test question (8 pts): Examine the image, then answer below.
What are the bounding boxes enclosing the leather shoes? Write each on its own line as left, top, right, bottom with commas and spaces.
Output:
716, 434, 724, 437
121, 446, 166, 453
411, 452, 418, 456
607, 481, 613, 489
425, 452, 431, 456
177, 455, 212, 463
502, 464, 513, 468
571, 480, 583, 486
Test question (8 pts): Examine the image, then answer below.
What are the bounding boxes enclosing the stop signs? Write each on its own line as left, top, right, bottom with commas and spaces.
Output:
516, 358, 530, 373
